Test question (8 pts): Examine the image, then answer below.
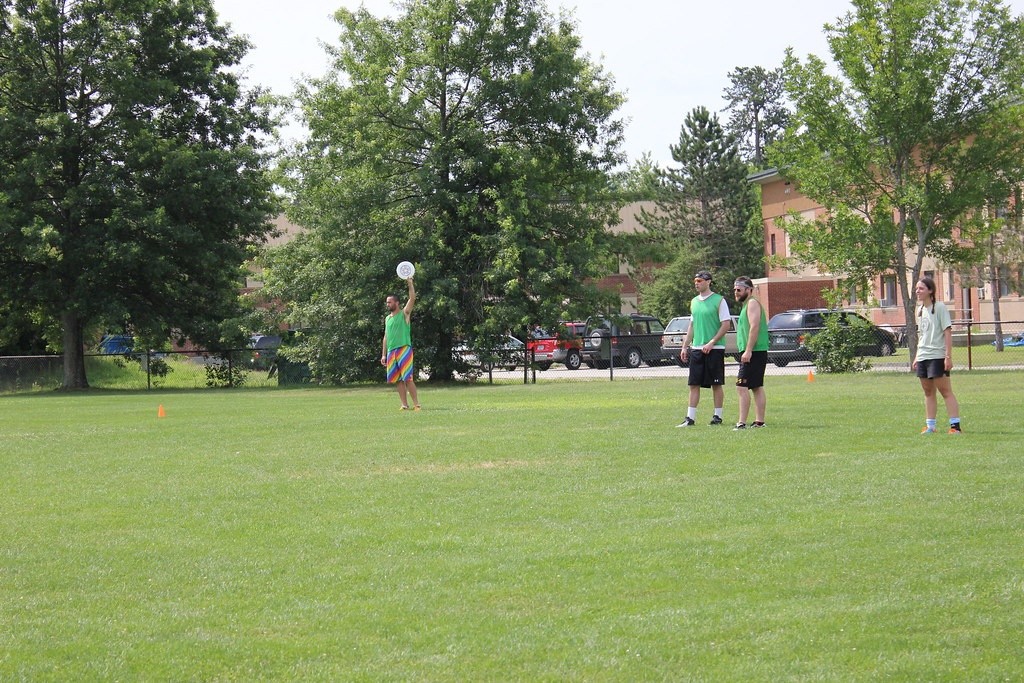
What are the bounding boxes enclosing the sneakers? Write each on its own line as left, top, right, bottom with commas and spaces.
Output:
675, 417, 695, 428
750, 421, 765, 429
708, 415, 722, 426
733, 422, 746, 430
947, 426, 961, 434
920, 426, 936, 434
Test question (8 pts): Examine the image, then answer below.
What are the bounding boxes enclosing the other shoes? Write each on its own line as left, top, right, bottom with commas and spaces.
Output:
399, 405, 421, 411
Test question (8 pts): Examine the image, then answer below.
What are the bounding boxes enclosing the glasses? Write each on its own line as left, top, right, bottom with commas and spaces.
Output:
734, 289, 741, 292
695, 279, 704, 283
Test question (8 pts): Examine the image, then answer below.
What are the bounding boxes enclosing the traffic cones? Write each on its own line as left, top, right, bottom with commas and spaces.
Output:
806, 371, 814, 382
157, 406, 167, 417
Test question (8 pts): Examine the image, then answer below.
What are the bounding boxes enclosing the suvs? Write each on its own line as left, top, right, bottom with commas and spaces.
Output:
661, 314, 741, 368
246, 332, 283, 372
579, 314, 675, 370
766, 308, 897, 366
525, 320, 587, 371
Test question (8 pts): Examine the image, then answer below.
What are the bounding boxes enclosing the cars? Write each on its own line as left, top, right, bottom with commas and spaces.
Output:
453, 335, 526, 372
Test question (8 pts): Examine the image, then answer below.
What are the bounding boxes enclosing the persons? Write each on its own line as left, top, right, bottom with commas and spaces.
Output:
912, 278, 962, 435
380, 276, 421, 413
731, 276, 769, 431
674, 270, 731, 427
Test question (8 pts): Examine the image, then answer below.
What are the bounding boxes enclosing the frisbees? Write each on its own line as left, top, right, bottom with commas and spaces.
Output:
396, 261, 415, 279
